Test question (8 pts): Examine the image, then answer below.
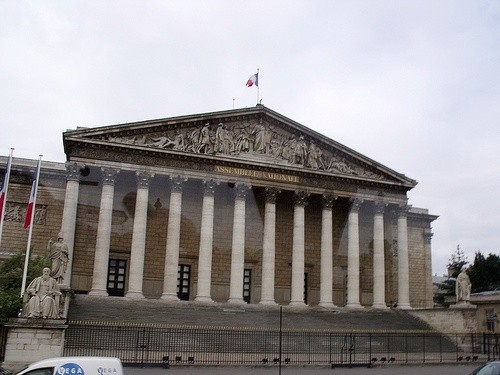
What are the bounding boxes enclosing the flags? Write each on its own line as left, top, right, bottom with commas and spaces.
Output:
245, 73, 258, 88
23, 179, 36, 229
0, 171, 7, 224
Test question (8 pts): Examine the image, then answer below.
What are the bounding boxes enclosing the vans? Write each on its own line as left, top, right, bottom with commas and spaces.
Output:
15, 356, 124, 375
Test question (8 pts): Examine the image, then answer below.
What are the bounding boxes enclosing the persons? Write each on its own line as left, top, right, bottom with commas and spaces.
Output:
47, 231, 70, 285
455, 265, 473, 303
109, 117, 384, 181
24, 267, 62, 319
